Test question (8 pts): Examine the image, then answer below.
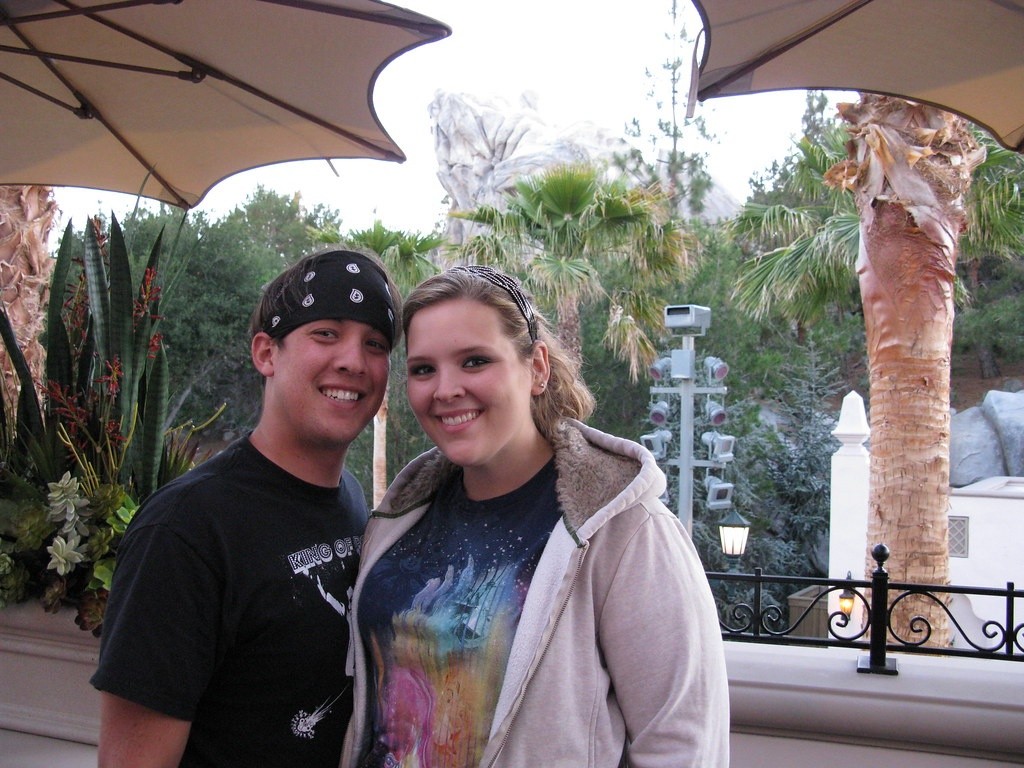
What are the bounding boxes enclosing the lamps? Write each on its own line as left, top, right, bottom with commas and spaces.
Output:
718, 502, 751, 571
839, 570, 856, 620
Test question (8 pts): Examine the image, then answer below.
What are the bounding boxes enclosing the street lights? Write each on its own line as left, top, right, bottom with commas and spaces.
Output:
714, 506, 751, 605
638, 303, 735, 543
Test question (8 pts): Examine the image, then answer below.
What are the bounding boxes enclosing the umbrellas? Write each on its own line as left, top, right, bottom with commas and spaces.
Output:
0, 0, 452, 210
686, 1, 1024, 153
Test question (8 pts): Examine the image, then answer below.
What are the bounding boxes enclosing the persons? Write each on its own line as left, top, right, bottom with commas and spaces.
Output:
339, 267, 732, 768
90, 247, 403, 768
